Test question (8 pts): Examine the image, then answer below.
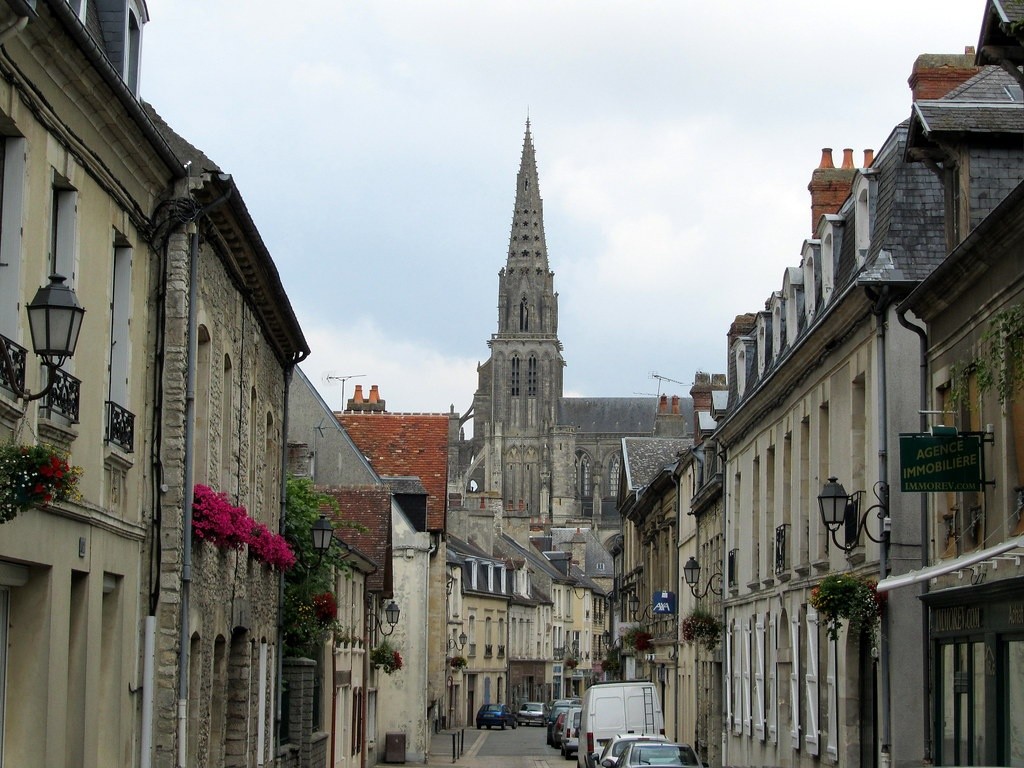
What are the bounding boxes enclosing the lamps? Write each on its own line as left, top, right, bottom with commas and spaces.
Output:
564, 641, 579, 655
286, 515, 333, 568
367, 600, 400, 637
602, 630, 623, 652
818, 477, 890, 550
449, 632, 467, 650
683, 556, 722, 600
628, 593, 655, 622
0, 276, 87, 401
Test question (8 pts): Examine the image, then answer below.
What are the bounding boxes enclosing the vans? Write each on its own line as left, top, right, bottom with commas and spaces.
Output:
575, 682, 666, 768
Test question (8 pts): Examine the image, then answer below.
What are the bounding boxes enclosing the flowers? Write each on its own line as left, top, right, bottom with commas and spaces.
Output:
371, 643, 402, 675
626, 628, 652, 651
810, 573, 880, 643
191, 484, 296, 569
451, 657, 469, 671
0, 440, 85, 525
683, 608, 723, 650
283, 583, 338, 661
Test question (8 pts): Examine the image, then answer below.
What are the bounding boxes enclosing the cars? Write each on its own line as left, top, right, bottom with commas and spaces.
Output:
602, 741, 709, 768
590, 734, 679, 768
547, 712, 567, 749
516, 702, 552, 728
476, 704, 518, 730
560, 707, 582, 760
547, 698, 582, 745
